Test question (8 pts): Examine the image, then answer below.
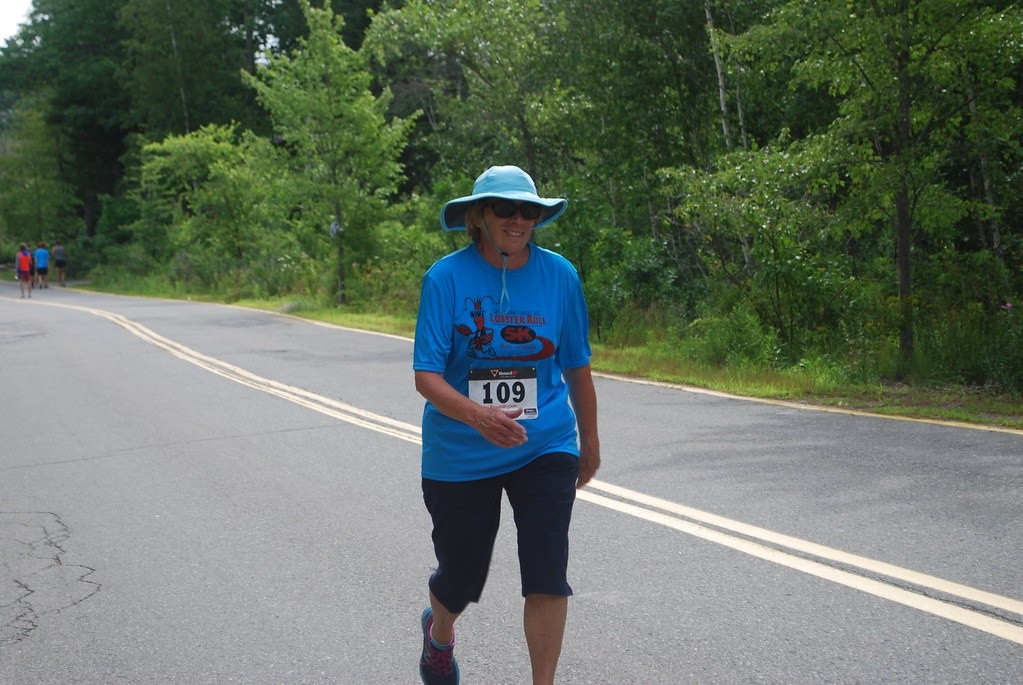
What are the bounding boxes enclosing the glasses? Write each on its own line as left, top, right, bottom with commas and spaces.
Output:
474, 200, 542, 220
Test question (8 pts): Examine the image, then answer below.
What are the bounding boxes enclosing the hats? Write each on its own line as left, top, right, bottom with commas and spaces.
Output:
440, 165, 569, 232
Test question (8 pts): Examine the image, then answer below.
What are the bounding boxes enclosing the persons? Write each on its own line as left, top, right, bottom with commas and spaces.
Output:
413, 165, 600, 685
16, 239, 69, 299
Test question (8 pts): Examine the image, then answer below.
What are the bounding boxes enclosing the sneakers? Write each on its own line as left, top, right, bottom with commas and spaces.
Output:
419, 608, 460, 685
